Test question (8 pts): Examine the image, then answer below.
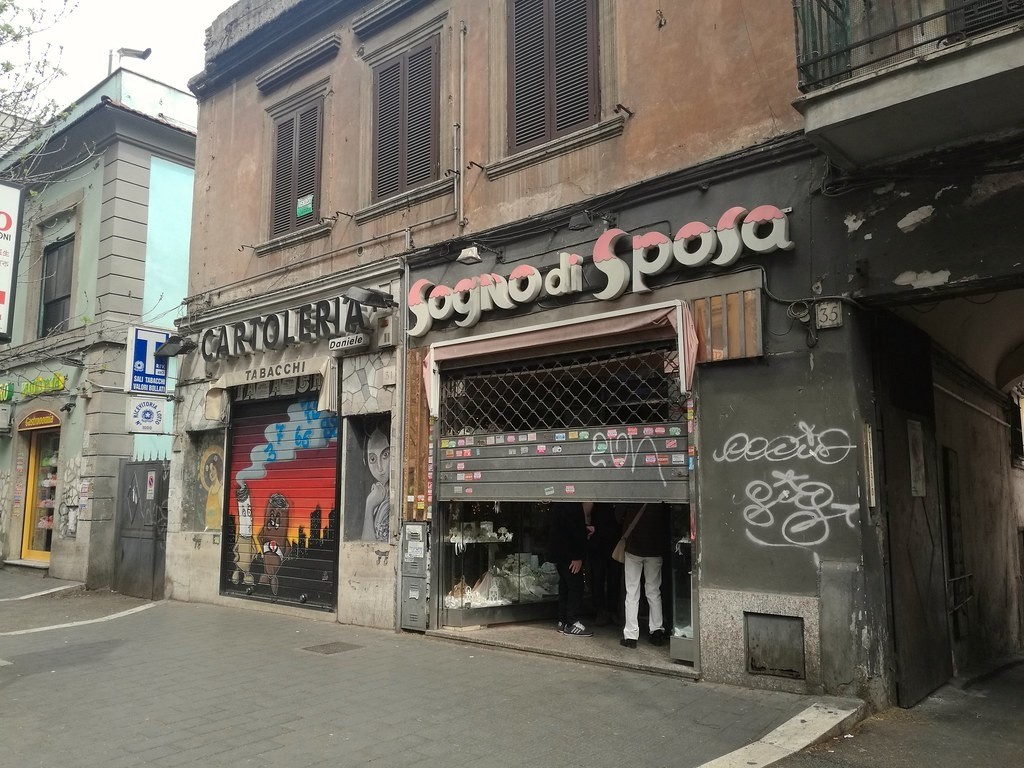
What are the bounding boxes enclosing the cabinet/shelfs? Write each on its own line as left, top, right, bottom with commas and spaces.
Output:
442, 501, 521, 611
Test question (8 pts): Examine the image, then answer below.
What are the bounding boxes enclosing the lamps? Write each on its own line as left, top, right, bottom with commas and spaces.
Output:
154, 335, 196, 357
567, 210, 617, 231
457, 241, 506, 265
343, 286, 398, 309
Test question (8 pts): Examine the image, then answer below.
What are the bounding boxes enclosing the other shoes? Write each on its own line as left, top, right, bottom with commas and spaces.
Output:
648, 629, 664, 646
620, 638, 636, 648
594, 610, 607, 626
606, 611, 623, 626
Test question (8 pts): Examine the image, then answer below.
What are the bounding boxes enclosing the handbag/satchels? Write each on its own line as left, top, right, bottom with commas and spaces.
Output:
612, 536, 626, 564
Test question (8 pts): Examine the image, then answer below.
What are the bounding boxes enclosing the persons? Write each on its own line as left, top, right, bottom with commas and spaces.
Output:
589, 503, 623, 627
543, 503, 595, 636
621, 503, 665, 648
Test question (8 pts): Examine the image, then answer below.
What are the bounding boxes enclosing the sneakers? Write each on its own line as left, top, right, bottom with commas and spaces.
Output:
557, 621, 565, 632
563, 621, 594, 637
